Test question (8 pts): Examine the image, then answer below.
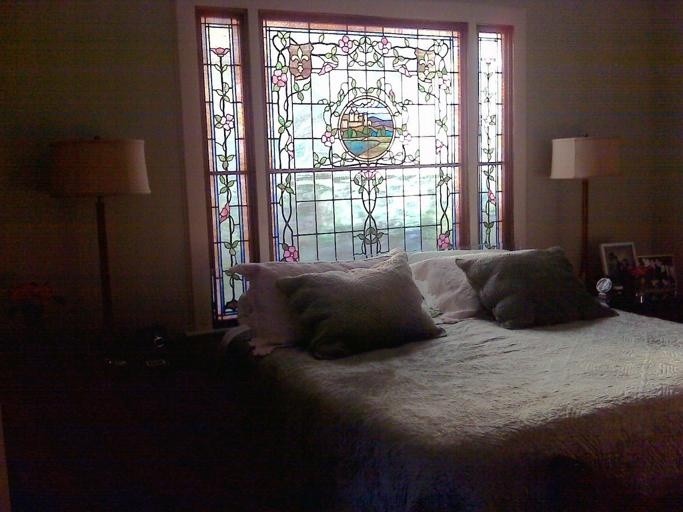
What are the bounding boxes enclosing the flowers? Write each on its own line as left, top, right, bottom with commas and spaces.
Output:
2, 275, 63, 312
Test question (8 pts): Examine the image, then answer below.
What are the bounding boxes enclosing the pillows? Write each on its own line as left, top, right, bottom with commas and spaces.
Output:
452, 242, 622, 330
410, 248, 544, 326
225, 256, 439, 363
274, 250, 449, 360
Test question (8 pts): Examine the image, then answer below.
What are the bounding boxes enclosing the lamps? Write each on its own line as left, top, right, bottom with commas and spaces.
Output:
46, 134, 155, 328
547, 132, 625, 282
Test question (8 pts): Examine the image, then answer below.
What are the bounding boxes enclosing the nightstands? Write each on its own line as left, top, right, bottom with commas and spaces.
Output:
597, 280, 683, 313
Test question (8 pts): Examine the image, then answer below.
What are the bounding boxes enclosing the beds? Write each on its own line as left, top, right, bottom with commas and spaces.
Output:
215, 296, 682, 509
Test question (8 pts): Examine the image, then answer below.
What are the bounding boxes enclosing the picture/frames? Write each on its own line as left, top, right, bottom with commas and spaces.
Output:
637, 253, 678, 293
597, 240, 640, 277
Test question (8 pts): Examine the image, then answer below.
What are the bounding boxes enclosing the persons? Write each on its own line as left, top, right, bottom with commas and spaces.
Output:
612, 258, 674, 281
